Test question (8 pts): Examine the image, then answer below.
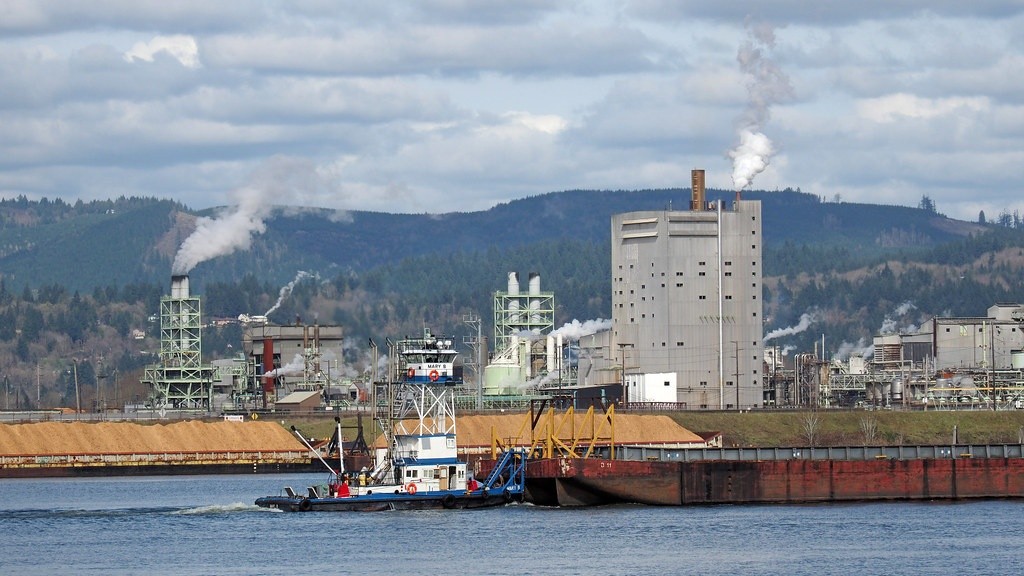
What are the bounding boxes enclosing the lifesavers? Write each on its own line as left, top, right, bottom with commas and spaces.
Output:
408, 367, 415, 379
429, 370, 440, 381
407, 483, 417, 495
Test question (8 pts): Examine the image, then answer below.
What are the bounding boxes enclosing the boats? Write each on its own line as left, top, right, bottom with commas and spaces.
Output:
255, 328, 526, 513
